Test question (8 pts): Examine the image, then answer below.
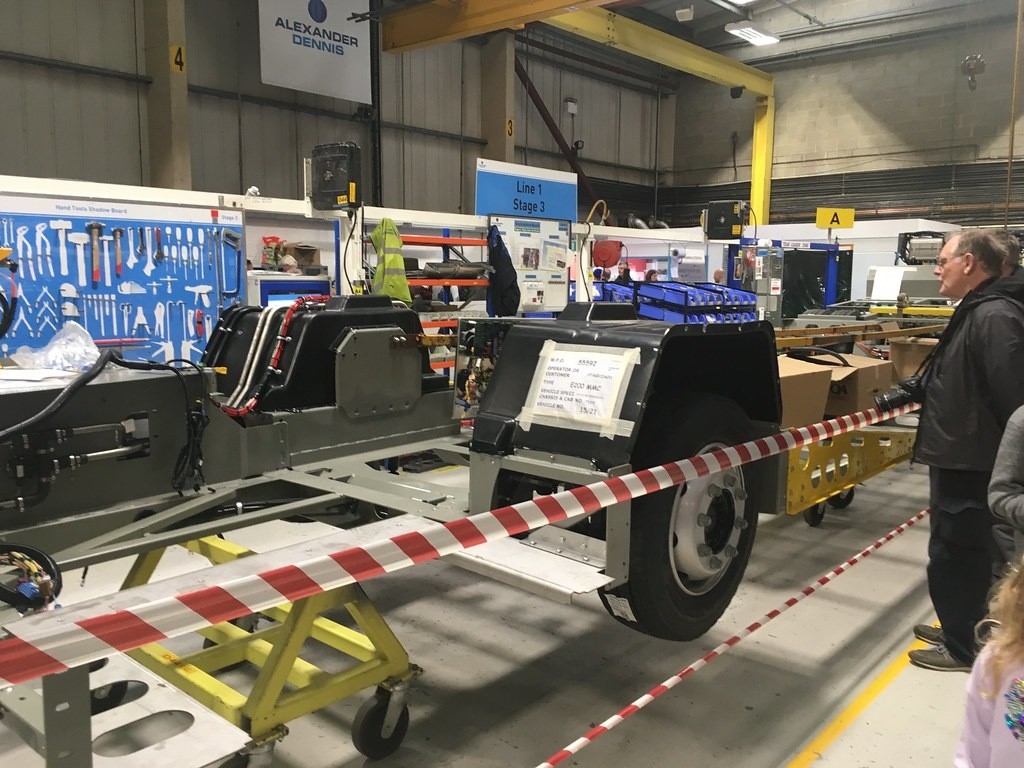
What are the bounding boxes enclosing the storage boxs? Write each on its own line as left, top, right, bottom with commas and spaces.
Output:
878, 320, 940, 388
283, 241, 320, 266
806, 351, 894, 416
776, 355, 833, 432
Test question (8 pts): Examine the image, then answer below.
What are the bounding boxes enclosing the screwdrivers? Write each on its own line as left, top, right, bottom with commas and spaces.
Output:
196, 310, 204, 343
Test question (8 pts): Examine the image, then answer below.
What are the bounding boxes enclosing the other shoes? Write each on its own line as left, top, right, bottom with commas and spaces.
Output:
914, 624, 947, 645
908, 645, 974, 672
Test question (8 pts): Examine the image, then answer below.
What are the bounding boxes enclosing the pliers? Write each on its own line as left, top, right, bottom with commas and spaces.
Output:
153, 227, 164, 262
136, 227, 146, 254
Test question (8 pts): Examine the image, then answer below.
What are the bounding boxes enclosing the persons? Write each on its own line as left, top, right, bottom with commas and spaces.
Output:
961, 566, 1024, 768
904, 229, 1024, 673
987, 406, 1023, 566
645, 270, 658, 280
987, 230, 1023, 279
713, 269, 726, 285
602, 269, 610, 281
614, 262, 631, 281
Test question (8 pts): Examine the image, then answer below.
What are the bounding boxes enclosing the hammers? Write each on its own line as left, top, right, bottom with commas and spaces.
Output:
111, 227, 126, 276
85, 220, 106, 282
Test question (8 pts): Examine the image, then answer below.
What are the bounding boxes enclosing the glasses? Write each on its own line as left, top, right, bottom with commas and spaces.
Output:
937, 253, 968, 267
